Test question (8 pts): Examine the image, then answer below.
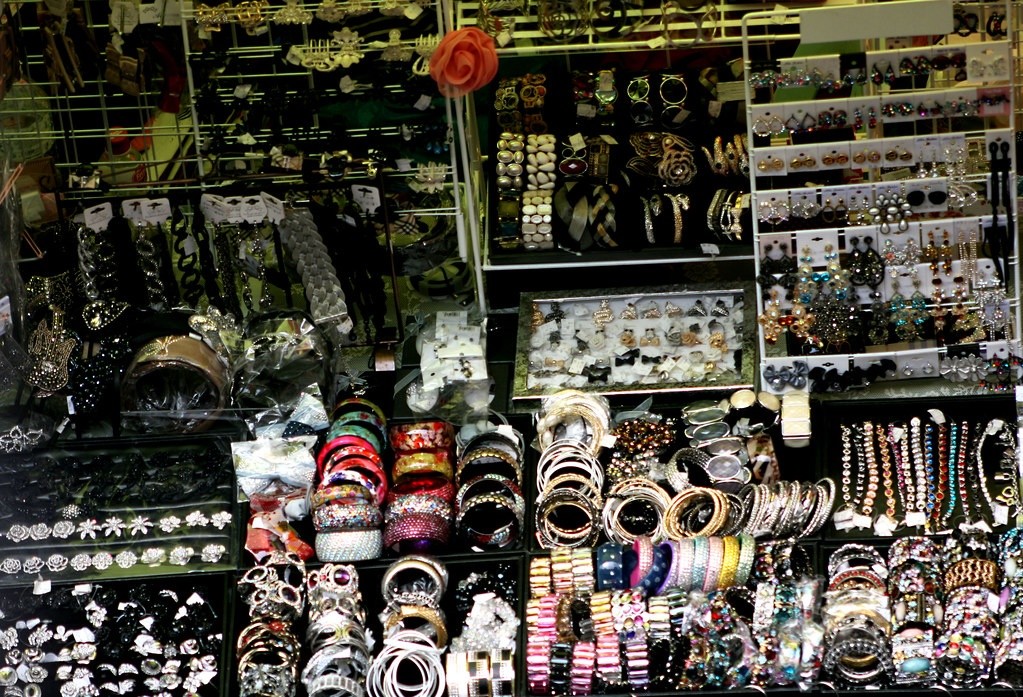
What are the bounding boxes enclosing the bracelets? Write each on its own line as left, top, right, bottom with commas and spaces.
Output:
244, 400, 1023, 697
491, 62, 752, 250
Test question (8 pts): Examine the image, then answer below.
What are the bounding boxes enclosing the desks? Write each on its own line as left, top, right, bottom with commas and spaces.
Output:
0, 312, 1023, 697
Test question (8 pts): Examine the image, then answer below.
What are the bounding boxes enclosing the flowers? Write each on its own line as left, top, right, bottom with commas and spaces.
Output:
427, 25, 499, 99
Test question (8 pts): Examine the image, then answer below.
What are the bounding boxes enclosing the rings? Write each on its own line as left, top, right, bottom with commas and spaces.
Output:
0, 451, 238, 697
529, 299, 730, 388
